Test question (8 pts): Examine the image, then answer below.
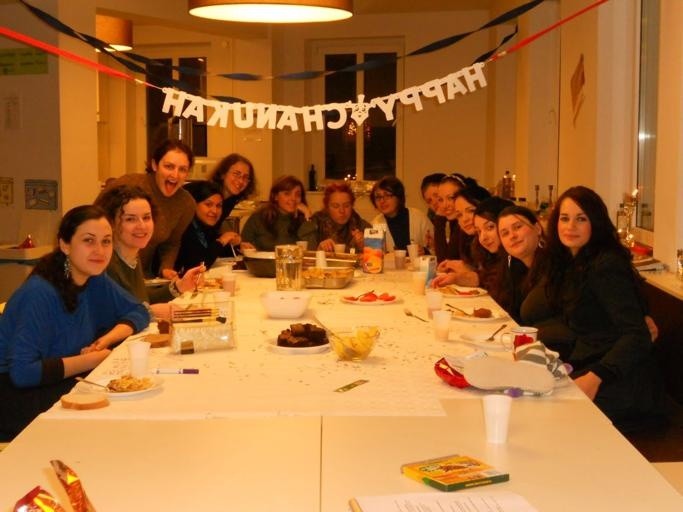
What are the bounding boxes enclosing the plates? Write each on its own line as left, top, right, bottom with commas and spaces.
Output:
340, 293, 399, 305
268, 338, 331, 354
89, 376, 166, 397
472, 335, 512, 349
442, 307, 500, 322
145, 279, 171, 286
438, 287, 488, 298
301, 267, 355, 288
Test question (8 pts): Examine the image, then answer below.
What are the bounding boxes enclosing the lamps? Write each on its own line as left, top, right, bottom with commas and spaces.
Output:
95, 14, 134, 53
186, 0, 354, 23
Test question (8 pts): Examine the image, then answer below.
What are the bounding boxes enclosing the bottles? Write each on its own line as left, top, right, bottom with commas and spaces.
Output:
362, 227, 385, 274
616, 203, 628, 238
502, 170, 511, 196
309, 165, 317, 192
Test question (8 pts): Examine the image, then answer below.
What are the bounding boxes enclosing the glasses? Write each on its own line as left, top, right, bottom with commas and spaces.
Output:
232, 171, 251, 183
374, 194, 394, 201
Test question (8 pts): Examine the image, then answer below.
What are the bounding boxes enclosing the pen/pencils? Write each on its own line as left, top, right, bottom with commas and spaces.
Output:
150, 368, 200, 375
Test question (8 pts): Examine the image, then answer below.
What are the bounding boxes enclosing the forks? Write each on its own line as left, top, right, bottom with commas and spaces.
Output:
403, 307, 428, 323
190, 261, 205, 299
485, 324, 507, 343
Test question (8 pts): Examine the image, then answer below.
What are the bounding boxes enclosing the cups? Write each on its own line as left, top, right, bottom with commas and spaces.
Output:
212, 292, 230, 303
221, 272, 237, 297
393, 250, 406, 271
333, 244, 346, 254
482, 394, 513, 445
296, 241, 308, 251
407, 244, 419, 261
427, 292, 443, 320
501, 326, 538, 352
432, 311, 452, 343
129, 342, 151, 380
412, 272, 427, 295
315, 250, 328, 268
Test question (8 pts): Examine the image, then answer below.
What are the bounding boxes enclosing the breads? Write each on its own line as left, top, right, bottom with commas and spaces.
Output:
61, 391, 110, 409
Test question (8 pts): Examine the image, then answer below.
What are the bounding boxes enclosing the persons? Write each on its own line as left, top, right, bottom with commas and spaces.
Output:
105, 140, 198, 281
240, 175, 318, 251
432, 205, 659, 340
432, 195, 516, 273
210, 153, 256, 231
440, 186, 490, 267
95, 186, 206, 305
428, 172, 476, 261
420, 172, 445, 221
313, 183, 374, 249
370, 176, 434, 254
0, 205, 152, 434
520, 184, 652, 402
176, 181, 242, 278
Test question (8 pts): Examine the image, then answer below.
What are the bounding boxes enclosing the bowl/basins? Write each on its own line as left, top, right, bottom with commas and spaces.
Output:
243, 251, 275, 278
259, 290, 314, 319
327, 326, 380, 361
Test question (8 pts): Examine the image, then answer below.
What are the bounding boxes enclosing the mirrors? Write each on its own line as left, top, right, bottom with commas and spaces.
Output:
632, 0, 661, 247
